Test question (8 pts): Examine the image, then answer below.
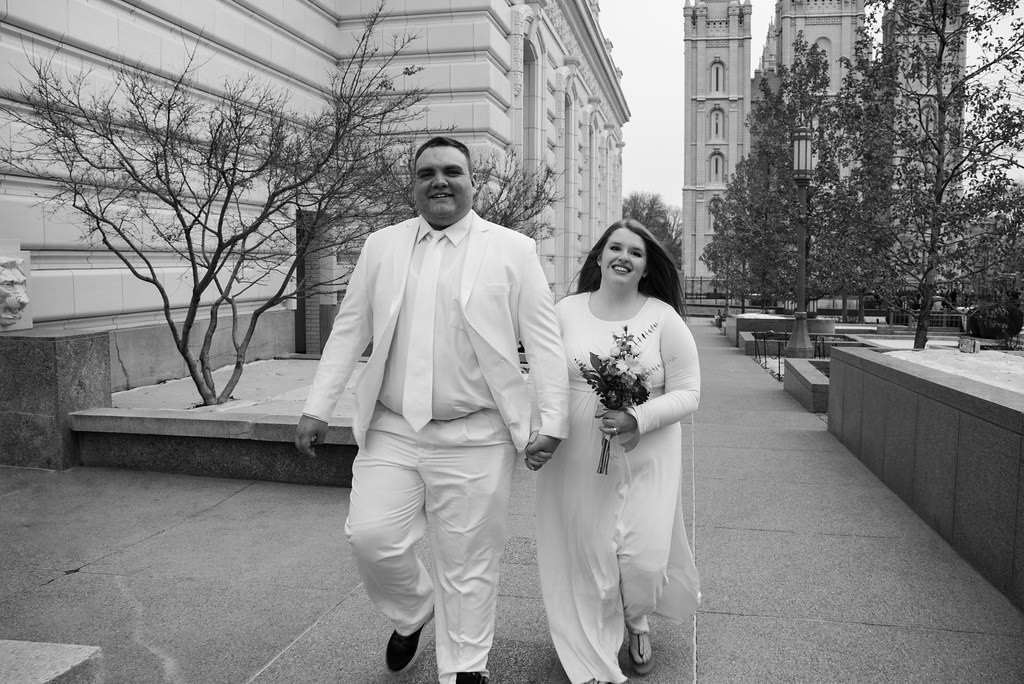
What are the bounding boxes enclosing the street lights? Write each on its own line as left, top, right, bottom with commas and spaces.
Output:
783, 120, 815, 358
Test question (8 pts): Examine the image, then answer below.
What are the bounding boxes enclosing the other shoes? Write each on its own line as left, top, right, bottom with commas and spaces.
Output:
384, 606, 434, 675
456, 672, 487, 684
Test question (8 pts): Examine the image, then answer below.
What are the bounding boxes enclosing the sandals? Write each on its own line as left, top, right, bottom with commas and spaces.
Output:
626, 624, 655, 674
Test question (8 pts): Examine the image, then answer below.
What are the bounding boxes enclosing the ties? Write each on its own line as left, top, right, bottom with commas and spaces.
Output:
402, 230, 446, 434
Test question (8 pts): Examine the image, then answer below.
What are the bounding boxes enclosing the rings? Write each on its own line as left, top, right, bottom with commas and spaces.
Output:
614, 427, 618, 435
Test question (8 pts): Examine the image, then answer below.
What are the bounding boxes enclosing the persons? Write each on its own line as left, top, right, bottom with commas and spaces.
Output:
521, 216, 702, 684
293, 135, 573, 681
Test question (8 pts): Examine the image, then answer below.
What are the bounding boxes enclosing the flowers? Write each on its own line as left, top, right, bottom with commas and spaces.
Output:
574, 323, 679, 477
